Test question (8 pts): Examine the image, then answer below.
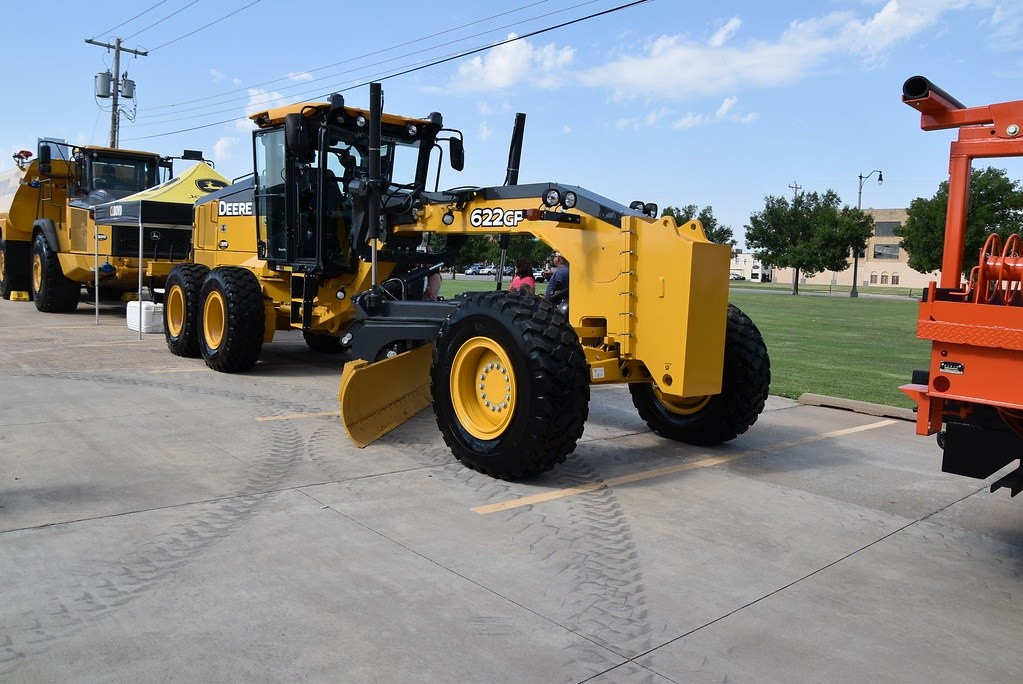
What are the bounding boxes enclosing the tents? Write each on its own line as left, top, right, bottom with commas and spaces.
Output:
94, 162, 234, 340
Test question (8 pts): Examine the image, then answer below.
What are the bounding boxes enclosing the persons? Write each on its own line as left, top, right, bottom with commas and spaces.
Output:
508, 258, 536, 292
546, 252, 568, 298
427, 247, 441, 298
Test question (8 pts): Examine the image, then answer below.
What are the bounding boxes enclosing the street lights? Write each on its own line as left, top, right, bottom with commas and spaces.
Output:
849, 170, 884, 299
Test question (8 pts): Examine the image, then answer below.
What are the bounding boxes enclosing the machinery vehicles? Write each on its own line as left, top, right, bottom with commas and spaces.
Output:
0, 137, 215, 315
900, 70, 1023, 501
160, 81, 771, 482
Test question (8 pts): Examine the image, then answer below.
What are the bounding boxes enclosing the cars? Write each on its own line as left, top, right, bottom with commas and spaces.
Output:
462, 262, 546, 283
